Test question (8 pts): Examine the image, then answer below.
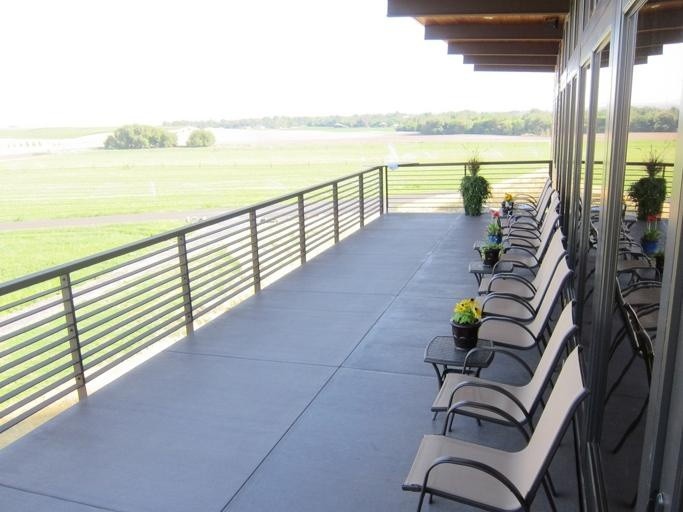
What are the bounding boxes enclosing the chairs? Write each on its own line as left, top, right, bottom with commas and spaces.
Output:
578, 198, 662, 454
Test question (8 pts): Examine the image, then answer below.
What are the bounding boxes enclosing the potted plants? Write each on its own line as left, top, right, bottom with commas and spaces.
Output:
628, 139, 667, 220
457, 143, 494, 216
